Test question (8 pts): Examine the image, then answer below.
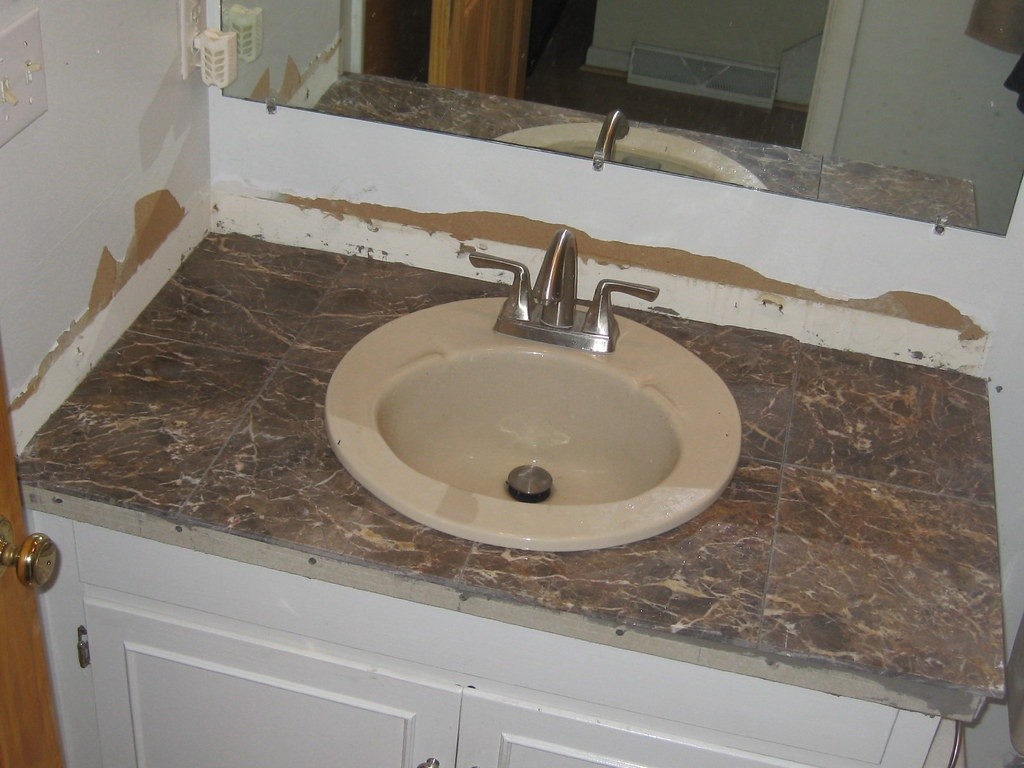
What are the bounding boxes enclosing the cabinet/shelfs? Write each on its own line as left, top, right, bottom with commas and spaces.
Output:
23, 510, 966, 768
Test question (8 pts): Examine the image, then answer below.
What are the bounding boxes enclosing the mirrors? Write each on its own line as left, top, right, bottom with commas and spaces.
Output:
348, 1, 865, 157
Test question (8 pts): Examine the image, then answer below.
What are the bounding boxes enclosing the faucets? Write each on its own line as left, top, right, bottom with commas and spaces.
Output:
530, 229, 580, 344
593, 109, 630, 161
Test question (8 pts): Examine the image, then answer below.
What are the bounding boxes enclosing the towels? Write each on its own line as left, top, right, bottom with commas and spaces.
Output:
961, 0, 1024, 113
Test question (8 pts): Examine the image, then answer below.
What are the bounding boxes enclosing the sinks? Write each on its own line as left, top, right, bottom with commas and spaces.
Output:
322, 295, 743, 554
494, 122, 768, 189
